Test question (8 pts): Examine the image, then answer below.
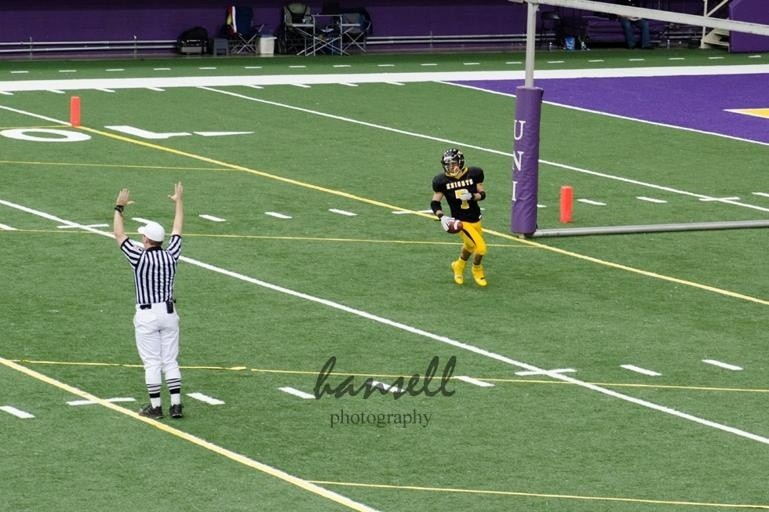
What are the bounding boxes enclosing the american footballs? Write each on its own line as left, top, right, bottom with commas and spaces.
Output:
447, 220, 463, 234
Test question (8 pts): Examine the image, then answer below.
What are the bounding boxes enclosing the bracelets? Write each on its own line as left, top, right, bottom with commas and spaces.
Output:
437, 213, 444, 218
114, 205, 126, 213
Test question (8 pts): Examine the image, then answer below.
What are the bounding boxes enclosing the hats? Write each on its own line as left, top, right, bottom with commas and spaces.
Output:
137, 221, 165, 242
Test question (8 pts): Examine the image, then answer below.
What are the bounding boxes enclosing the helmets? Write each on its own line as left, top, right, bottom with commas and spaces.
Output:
440, 148, 464, 177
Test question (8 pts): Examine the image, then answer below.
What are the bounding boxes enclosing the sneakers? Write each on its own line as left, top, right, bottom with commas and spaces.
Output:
451, 261, 464, 285
170, 403, 182, 417
471, 265, 488, 287
140, 404, 165, 420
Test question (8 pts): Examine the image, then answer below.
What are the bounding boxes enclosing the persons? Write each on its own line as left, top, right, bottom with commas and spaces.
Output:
430, 147, 489, 287
558, 3, 593, 51
618, 0, 655, 50
113, 180, 185, 421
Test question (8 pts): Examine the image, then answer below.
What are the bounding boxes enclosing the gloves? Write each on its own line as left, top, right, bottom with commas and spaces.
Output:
441, 215, 455, 232
459, 192, 472, 201
